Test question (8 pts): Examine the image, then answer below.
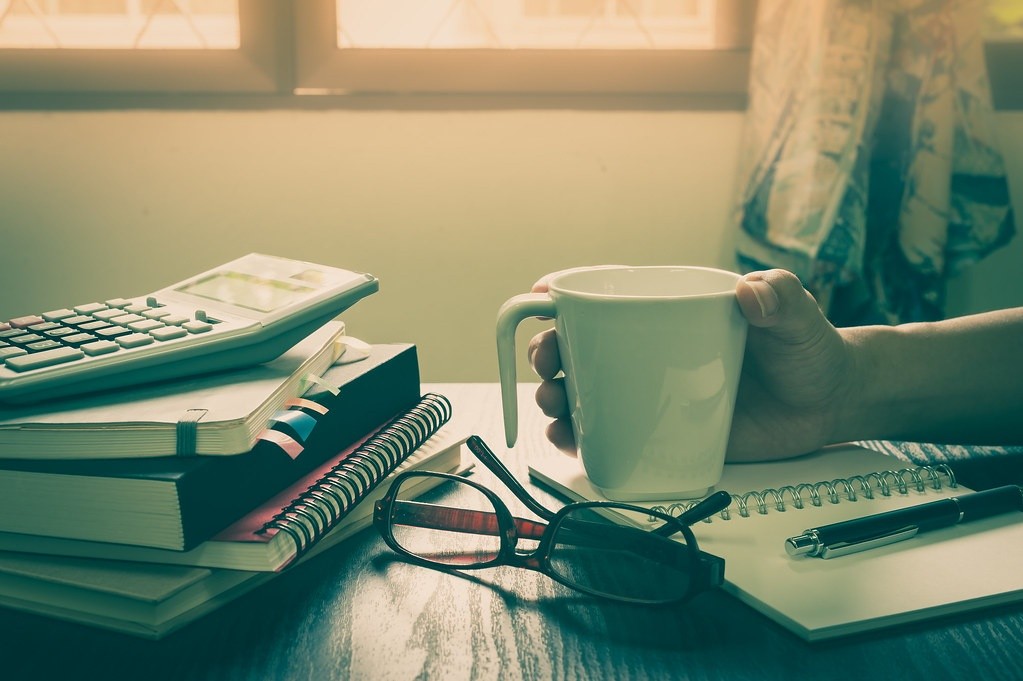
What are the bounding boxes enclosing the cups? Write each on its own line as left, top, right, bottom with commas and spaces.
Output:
495, 264, 751, 504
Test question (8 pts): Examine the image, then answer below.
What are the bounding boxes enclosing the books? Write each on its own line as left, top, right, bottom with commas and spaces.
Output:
0, 319, 344, 461
0, 392, 480, 645
0, 343, 420, 554
528, 446, 1023, 639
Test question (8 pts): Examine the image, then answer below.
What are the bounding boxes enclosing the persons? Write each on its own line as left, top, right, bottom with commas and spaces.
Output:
527, 264, 1023, 462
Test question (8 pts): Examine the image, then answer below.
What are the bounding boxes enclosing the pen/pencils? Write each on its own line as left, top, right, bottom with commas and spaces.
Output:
784, 483, 1023, 560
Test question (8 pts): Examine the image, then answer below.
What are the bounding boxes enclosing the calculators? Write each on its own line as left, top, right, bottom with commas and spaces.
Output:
1, 255, 380, 432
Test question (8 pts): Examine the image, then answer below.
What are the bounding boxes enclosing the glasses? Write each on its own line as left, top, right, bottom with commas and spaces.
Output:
372, 435, 732, 607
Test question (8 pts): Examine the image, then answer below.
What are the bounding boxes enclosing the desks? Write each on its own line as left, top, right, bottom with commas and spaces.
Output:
0, 382, 1023, 681
